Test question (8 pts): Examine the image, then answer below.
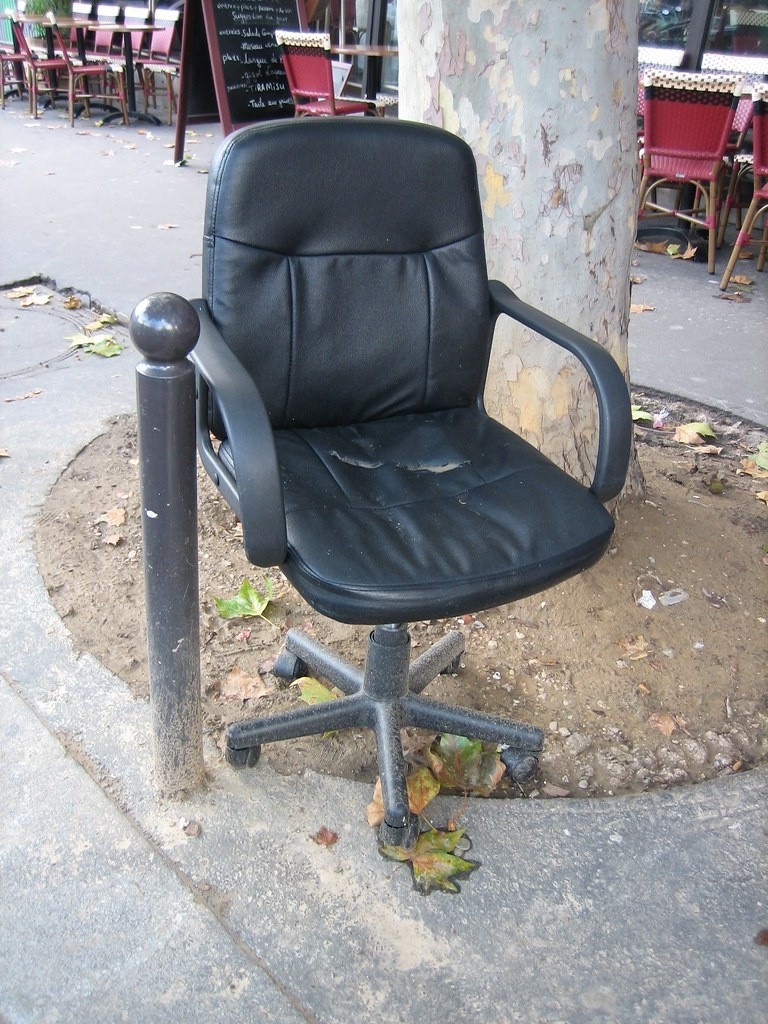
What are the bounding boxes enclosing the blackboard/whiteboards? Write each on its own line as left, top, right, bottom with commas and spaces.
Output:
177, 0, 308, 131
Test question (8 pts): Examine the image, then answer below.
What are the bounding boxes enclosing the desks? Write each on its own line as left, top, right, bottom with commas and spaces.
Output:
636, 79, 757, 264
16, 18, 80, 110
328, 44, 399, 117
42, 19, 121, 118
88, 24, 167, 126
0, 9, 46, 100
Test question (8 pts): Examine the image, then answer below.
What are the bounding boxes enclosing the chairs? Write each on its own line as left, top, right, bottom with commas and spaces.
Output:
0, 0, 768, 847
